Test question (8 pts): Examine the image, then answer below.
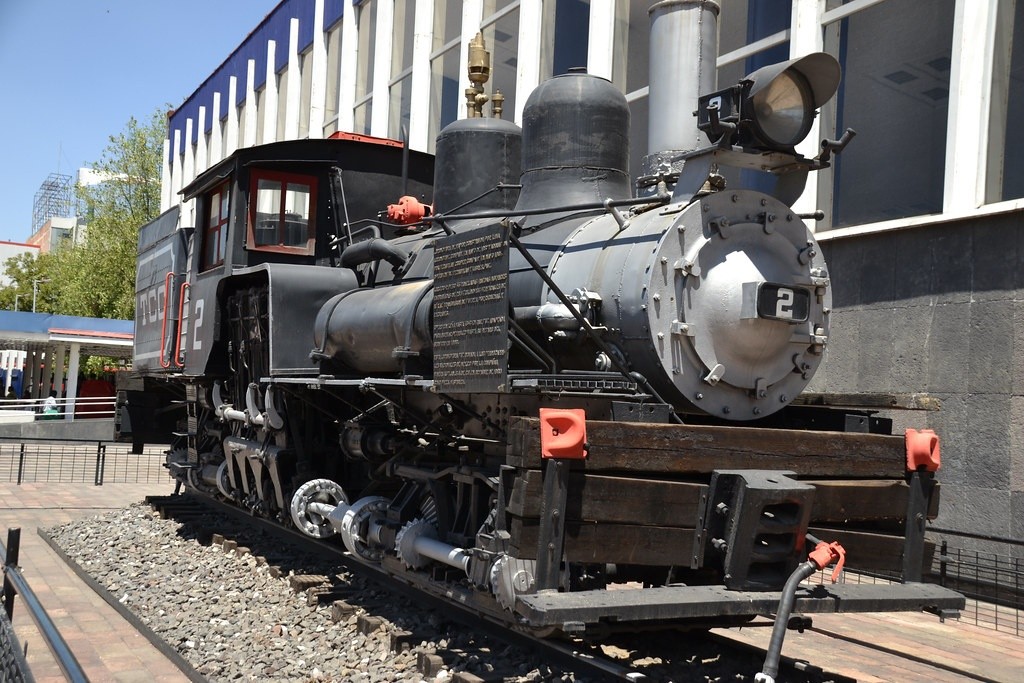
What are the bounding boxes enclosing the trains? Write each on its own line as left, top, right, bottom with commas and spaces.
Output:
110, 27, 966, 683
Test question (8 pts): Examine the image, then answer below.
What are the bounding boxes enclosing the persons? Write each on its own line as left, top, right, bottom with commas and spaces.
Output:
43, 390, 59, 419
23, 390, 31, 399
7, 386, 18, 399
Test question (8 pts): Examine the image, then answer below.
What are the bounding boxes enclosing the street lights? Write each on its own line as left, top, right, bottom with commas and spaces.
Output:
14, 294, 31, 313
32, 277, 52, 313
